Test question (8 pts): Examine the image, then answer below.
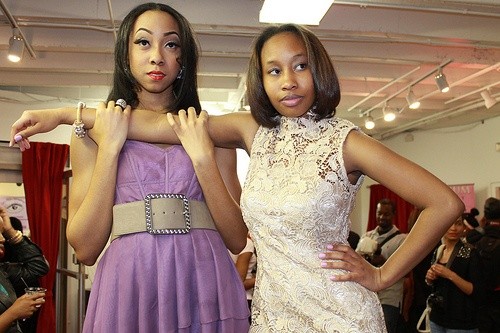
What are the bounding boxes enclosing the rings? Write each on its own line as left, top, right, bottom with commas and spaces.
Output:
116, 99, 126, 108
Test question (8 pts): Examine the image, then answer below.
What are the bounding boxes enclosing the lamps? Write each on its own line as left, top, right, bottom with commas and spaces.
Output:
434, 66, 449, 94
7, 26, 24, 62
382, 101, 395, 123
364, 112, 376, 130
479, 88, 498, 110
405, 87, 421, 109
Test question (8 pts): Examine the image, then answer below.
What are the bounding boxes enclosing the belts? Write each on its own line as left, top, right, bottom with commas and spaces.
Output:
109, 192, 218, 243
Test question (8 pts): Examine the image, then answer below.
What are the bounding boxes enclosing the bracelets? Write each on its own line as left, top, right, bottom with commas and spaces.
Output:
75, 102, 86, 137
11, 230, 22, 244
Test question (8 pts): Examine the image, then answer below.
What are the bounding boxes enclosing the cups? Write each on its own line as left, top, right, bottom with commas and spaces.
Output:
24, 287, 47, 308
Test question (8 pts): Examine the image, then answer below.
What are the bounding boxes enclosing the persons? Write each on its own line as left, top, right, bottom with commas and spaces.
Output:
0, 206, 50, 333
407, 198, 500, 333
0, 196, 28, 228
227, 233, 257, 326
354, 199, 410, 333
9, 24, 466, 333
66, 3, 252, 333
